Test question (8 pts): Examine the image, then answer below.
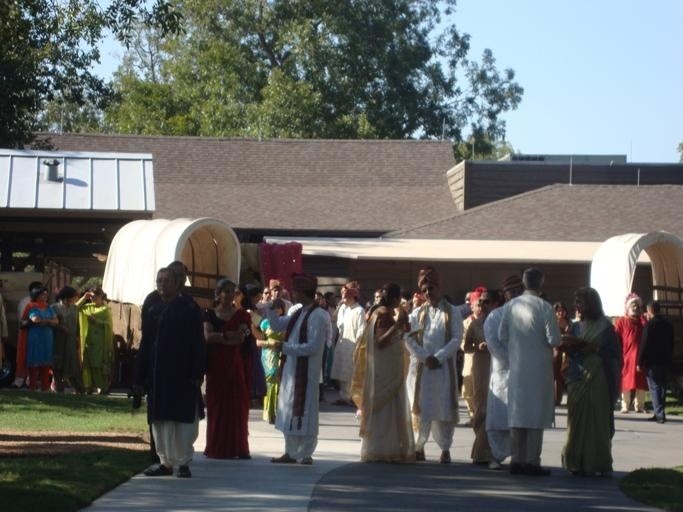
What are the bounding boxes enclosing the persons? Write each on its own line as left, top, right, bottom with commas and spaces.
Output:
464, 290, 501, 470
350, 283, 416, 463
404, 265, 463, 463
560, 287, 621, 474
498, 265, 560, 476
204, 279, 252, 460
141, 259, 202, 462
483, 274, 524, 461
263, 271, 327, 464
234, 281, 670, 423
1, 273, 113, 396
132, 267, 204, 478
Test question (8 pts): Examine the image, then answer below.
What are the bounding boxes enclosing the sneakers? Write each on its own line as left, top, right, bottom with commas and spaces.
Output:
509, 464, 550, 475
271, 453, 294, 463
177, 466, 191, 477
144, 465, 172, 476
